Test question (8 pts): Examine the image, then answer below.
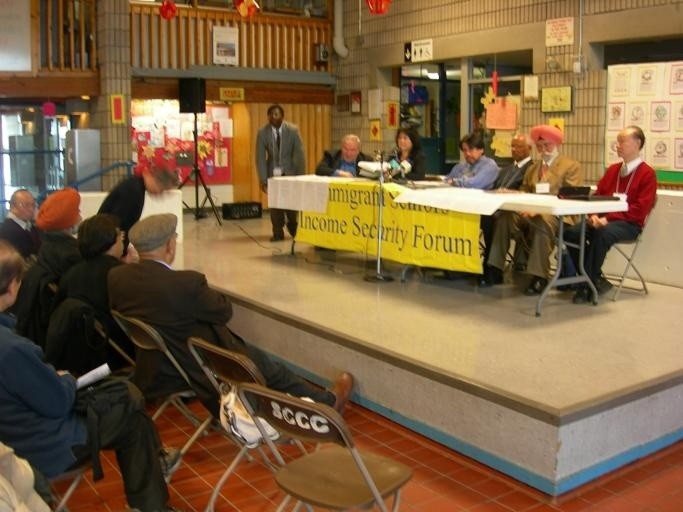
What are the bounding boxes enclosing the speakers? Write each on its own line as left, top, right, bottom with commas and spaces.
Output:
314, 44, 331, 62
178, 77, 206, 113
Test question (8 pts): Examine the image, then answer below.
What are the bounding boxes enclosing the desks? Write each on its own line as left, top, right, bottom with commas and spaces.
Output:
266, 173, 629, 316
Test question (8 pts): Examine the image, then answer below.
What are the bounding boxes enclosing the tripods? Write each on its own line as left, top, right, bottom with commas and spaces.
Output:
177, 113, 223, 227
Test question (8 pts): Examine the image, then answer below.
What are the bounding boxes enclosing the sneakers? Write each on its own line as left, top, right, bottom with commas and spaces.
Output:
160, 447, 182, 473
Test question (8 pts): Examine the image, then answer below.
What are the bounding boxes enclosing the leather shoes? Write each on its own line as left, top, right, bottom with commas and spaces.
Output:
327, 371, 352, 415
269, 233, 284, 242
590, 276, 612, 300
524, 277, 548, 294
479, 269, 504, 285
572, 283, 593, 303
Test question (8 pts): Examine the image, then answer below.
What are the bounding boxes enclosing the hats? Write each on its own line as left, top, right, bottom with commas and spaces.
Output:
128, 213, 177, 251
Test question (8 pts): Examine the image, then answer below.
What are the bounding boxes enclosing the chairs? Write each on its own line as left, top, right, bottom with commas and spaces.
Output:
1, 453, 98, 512
164, 336, 302, 511
612, 195, 659, 302
82, 313, 140, 369
234, 381, 413, 512
109, 309, 220, 435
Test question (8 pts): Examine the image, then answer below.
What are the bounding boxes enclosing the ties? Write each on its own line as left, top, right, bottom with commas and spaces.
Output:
502, 166, 518, 187
537, 161, 547, 183
275, 129, 280, 152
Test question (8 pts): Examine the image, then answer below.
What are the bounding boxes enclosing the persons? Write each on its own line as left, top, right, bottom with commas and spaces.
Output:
107, 214, 353, 423
0, 243, 179, 511
562, 126, 656, 303
478, 125, 583, 296
383, 128, 427, 182
93, 167, 175, 237
37, 187, 80, 274
64, 215, 128, 354
313, 135, 374, 250
5, 190, 39, 256
254, 106, 305, 242
434, 134, 534, 279
416, 134, 498, 269
